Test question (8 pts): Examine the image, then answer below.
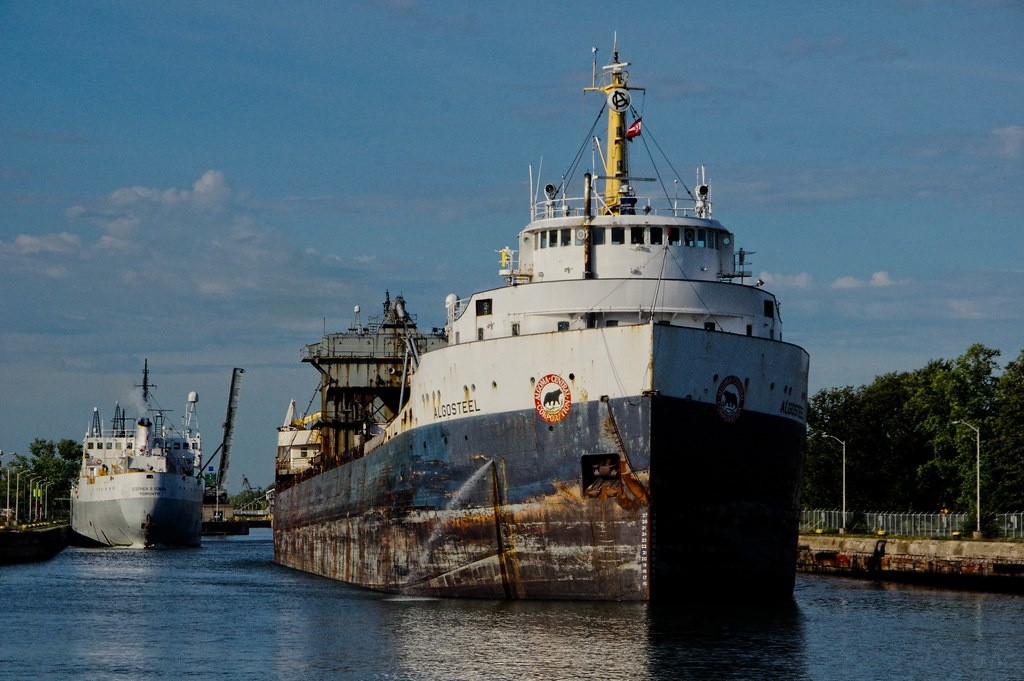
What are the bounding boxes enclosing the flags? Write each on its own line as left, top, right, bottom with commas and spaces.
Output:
625, 116, 642, 142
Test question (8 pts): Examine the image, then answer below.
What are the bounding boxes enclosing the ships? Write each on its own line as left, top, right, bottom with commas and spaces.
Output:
271, 31, 808, 607
74, 360, 206, 553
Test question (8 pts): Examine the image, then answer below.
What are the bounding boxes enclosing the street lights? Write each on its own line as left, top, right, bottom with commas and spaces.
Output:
24, 471, 36, 524
30, 476, 54, 523
14, 469, 30, 524
5, 463, 22, 527
